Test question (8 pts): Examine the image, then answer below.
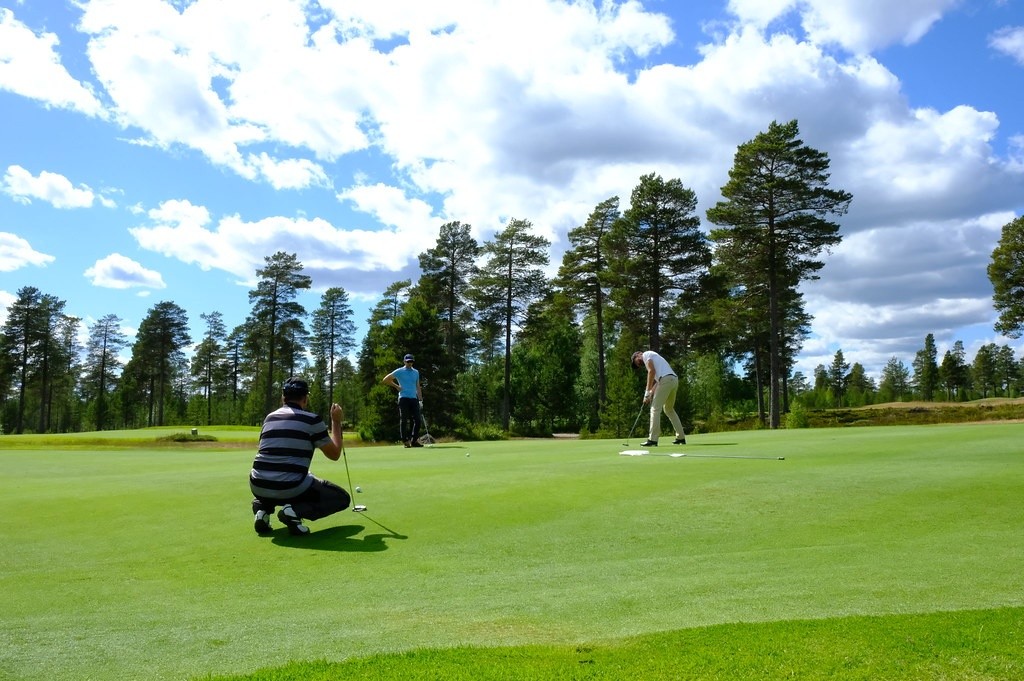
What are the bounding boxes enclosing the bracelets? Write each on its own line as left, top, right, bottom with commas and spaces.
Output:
419, 399, 423, 402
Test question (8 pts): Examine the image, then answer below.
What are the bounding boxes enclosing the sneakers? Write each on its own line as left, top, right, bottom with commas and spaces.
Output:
277, 503, 310, 535
254, 509, 273, 536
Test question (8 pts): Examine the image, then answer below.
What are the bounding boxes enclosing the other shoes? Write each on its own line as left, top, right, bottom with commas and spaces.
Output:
640, 440, 657, 446
411, 440, 424, 447
404, 441, 411, 448
672, 438, 686, 444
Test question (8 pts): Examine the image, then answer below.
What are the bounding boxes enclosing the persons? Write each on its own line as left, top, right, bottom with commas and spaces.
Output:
248, 376, 350, 536
630, 350, 687, 447
383, 354, 424, 447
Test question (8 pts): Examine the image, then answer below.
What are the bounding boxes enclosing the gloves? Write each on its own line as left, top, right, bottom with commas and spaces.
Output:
419, 400, 424, 410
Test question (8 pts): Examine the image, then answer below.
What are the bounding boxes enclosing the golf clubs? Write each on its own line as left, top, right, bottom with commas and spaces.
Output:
421, 409, 434, 448
622, 404, 645, 446
339, 426, 367, 512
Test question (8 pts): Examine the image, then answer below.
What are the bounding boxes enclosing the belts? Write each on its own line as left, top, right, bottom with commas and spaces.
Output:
659, 374, 675, 382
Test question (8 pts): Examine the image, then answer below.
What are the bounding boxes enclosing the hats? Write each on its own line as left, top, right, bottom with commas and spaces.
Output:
631, 351, 641, 369
283, 377, 312, 397
404, 354, 415, 361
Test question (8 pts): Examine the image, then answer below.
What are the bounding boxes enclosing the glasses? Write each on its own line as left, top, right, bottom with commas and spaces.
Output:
405, 361, 414, 363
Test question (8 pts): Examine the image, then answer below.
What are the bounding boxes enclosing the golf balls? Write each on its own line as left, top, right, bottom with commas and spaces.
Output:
356, 486, 361, 492
466, 453, 470, 457
